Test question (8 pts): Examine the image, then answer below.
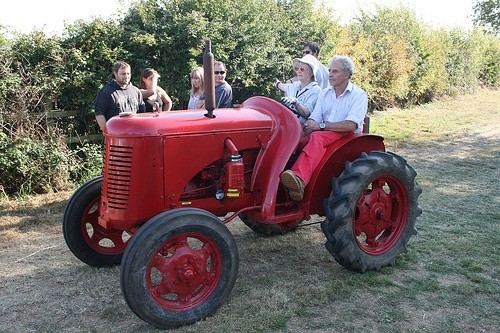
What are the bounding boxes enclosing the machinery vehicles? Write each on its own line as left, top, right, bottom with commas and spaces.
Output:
63, 41, 422, 329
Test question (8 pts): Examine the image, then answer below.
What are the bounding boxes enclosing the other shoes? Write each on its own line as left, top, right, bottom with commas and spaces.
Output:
281, 170, 306, 202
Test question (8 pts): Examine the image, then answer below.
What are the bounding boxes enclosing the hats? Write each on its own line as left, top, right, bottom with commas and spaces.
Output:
292, 54, 321, 82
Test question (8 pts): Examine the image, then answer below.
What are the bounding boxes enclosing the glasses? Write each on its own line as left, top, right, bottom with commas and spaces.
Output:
303, 50, 310, 55
214, 70, 225, 76
294, 67, 306, 72
